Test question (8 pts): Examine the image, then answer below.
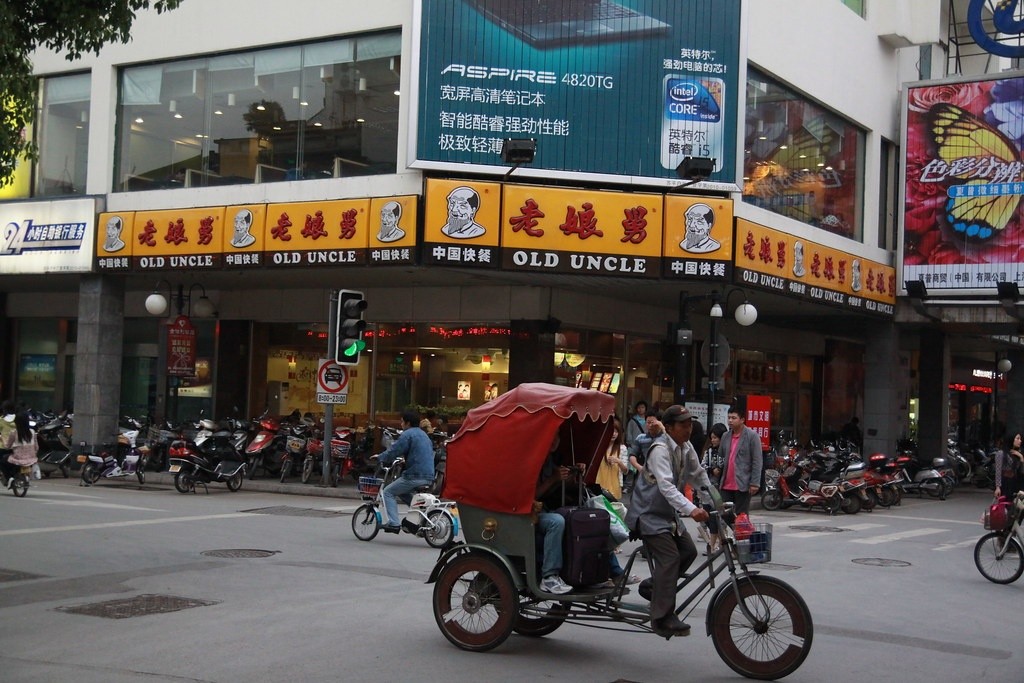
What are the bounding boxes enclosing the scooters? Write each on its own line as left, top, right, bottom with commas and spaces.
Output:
760, 427, 1008, 517
974, 488, 1024, 585
0, 407, 455, 501
351, 455, 461, 549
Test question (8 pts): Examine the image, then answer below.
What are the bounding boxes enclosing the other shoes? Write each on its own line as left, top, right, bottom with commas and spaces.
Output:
698, 536, 706, 542
385, 524, 401, 534
702, 550, 716, 555
612, 574, 641, 586
639, 579, 653, 601
7, 478, 15, 489
651, 613, 691, 635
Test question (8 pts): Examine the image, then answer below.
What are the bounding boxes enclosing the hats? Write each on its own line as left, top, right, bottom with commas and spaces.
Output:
662, 406, 698, 424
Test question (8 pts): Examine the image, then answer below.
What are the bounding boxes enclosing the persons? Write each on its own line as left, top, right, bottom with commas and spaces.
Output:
532, 426, 641, 595
700, 423, 728, 556
595, 419, 630, 500
419, 410, 435, 433
840, 416, 860, 445
718, 404, 763, 560
625, 404, 722, 632
678, 420, 705, 517
628, 411, 666, 485
285, 157, 307, 180
978, 410, 1006, 446
622, 400, 649, 494
697, 436, 712, 542
995, 430, 1024, 551
369, 407, 435, 535
819, 207, 850, 237
485, 383, 497, 400
458, 384, 469, 398
0, 400, 38, 489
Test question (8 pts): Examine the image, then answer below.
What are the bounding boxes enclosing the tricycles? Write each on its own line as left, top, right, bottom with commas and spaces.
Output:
425, 383, 814, 680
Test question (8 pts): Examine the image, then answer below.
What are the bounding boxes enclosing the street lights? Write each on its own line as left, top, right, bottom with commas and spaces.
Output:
706, 286, 758, 443
144, 277, 216, 423
992, 343, 1012, 448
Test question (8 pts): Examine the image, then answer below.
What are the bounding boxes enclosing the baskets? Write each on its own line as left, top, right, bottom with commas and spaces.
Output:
286, 435, 306, 454
359, 476, 383, 500
330, 439, 351, 458
730, 522, 773, 564
765, 469, 781, 485
385, 439, 399, 451
307, 438, 324, 456
135, 427, 173, 454
984, 505, 1009, 531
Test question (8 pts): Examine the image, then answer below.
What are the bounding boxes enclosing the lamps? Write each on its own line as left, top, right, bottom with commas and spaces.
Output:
359, 76, 367, 92
903, 278, 929, 298
227, 94, 235, 106
287, 354, 298, 379
293, 86, 299, 100
500, 137, 537, 176
412, 350, 424, 374
80, 110, 87, 123
481, 354, 491, 374
994, 280, 1022, 303
170, 100, 177, 113
673, 154, 716, 192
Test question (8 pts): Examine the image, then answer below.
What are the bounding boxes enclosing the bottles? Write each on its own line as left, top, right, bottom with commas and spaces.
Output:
450, 505, 462, 518
735, 530, 768, 563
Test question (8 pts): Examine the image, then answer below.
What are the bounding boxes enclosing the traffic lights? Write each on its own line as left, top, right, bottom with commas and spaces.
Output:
335, 289, 369, 366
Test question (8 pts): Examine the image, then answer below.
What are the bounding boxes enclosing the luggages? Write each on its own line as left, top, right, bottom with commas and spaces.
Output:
555, 464, 612, 587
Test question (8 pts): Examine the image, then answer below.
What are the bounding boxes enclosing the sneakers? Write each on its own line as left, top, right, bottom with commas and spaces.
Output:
540, 574, 575, 594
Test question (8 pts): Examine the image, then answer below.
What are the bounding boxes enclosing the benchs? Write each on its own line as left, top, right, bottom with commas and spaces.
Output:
124, 157, 369, 192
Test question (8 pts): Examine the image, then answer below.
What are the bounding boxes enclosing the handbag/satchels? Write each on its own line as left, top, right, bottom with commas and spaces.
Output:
988, 495, 1012, 530
583, 486, 631, 550
31, 462, 42, 479
